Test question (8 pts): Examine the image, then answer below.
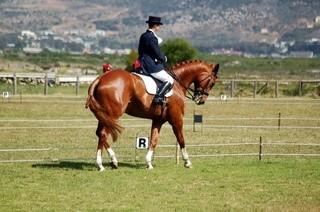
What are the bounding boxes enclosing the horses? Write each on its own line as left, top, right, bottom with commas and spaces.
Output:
85, 60, 220, 171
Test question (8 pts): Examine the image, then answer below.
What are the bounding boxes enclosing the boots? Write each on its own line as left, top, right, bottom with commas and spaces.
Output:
152, 81, 171, 106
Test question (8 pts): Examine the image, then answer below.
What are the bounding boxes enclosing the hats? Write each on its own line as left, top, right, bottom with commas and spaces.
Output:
146, 16, 163, 25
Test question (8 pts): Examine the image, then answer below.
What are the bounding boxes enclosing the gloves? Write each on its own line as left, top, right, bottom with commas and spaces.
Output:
165, 56, 167, 62
155, 58, 157, 62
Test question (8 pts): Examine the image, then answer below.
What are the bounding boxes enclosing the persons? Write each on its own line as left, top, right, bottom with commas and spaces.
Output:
138, 16, 175, 106
